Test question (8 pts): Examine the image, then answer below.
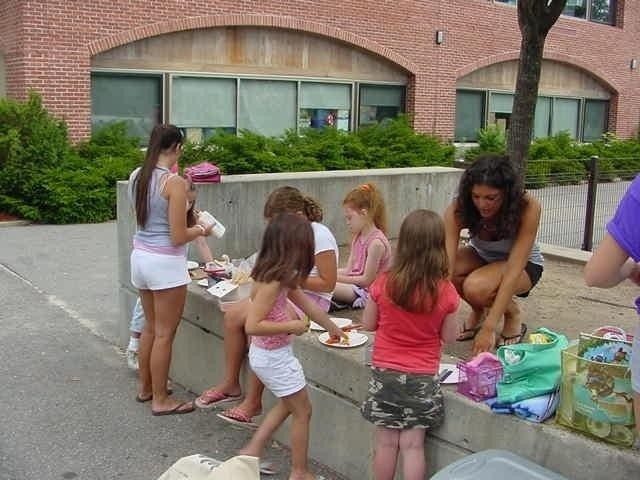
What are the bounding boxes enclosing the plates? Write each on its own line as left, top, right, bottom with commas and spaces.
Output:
437, 362, 469, 384
187, 260, 199, 270
309, 318, 353, 331
318, 331, 368, 347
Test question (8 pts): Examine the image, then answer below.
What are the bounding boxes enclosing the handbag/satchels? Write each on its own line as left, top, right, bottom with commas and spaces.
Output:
184, 164, 221, 183
496, 327, 568, 404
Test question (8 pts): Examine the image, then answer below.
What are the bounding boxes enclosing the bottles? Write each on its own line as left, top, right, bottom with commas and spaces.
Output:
197, 211, 226, 239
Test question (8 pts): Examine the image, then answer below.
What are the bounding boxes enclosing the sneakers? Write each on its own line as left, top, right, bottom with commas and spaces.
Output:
127, 346, 140, 371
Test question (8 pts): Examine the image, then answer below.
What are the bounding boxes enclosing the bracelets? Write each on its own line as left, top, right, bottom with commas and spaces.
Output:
194, 223, 209, 239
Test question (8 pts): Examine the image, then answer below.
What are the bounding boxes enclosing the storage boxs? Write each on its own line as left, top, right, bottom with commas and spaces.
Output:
428, 448, 567, 479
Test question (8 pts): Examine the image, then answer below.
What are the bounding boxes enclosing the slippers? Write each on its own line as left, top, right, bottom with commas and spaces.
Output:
456, 320, 481, 341
136, 383, 172, 401
152, 400, 192, 416
496, 323, 527, 347
194, 389, 242, 408
261, 461, 276, 475
216, 408, 260, 428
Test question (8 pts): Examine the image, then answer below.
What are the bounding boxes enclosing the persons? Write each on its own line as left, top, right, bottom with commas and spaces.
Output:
443, 154, 545, 357
362, 207, 463, 479
583, 171, 640, 445
326, 183, 393, 311
125, 175, 212, 371
128, 121, 215, 417
237, 209, 349, 479
194, 186, 339, 423
